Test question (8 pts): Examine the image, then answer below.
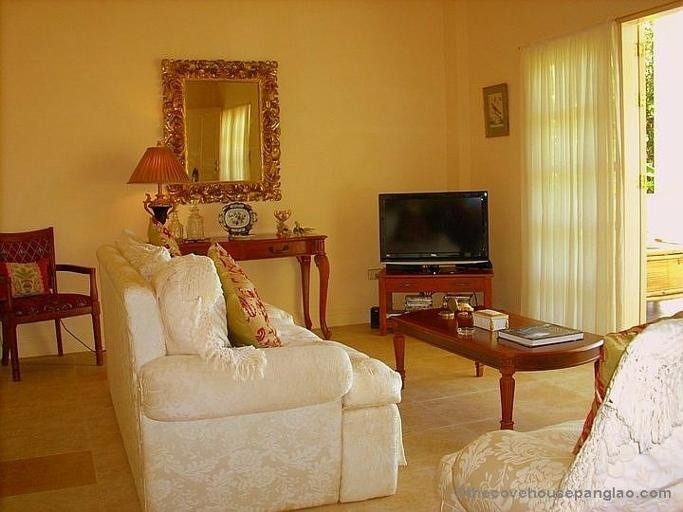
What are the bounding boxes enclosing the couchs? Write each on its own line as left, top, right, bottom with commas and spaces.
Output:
99, 238, 401, 510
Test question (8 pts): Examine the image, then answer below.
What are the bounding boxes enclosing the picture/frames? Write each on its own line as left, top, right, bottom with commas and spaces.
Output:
482, 83, 510, 138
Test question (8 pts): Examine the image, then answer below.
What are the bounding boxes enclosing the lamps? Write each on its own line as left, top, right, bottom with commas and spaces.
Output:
127, 140, 192, 226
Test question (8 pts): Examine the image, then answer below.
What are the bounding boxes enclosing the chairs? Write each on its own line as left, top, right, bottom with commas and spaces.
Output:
0, 226, 104, 382
437, 319, 683, 511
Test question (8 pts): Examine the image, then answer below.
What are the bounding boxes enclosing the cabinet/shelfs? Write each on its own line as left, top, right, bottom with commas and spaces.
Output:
376, 268, 495, 337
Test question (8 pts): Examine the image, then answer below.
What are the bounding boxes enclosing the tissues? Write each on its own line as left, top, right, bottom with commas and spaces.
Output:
472, 308, 510, 331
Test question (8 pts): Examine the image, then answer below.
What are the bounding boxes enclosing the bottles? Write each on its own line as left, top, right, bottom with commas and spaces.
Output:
168, 196, 203, 246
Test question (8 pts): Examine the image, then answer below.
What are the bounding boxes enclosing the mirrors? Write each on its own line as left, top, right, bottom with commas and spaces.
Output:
162, 59, 282, 204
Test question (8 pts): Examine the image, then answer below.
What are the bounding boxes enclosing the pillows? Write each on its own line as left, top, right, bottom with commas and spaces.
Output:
1, 258, 50, 298
572, 310, 683, 456
117, 234, 283, 355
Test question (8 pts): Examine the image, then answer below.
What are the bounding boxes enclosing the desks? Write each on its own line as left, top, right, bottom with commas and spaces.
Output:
164, 234, 331, 339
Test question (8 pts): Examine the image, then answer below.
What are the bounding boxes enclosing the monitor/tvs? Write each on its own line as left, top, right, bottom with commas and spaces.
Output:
379, 191, 489, 272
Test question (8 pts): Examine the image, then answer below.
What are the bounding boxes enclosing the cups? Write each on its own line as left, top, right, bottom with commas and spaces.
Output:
455, 311, 475, 336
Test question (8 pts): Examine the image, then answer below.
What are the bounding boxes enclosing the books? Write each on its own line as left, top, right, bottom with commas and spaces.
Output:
497, 322, 584, 347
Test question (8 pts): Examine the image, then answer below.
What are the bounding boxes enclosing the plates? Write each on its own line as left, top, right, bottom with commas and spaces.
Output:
217, 202, 257, 233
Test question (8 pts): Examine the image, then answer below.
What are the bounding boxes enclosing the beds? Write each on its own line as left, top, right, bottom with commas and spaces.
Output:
388, 305, 605, 430
